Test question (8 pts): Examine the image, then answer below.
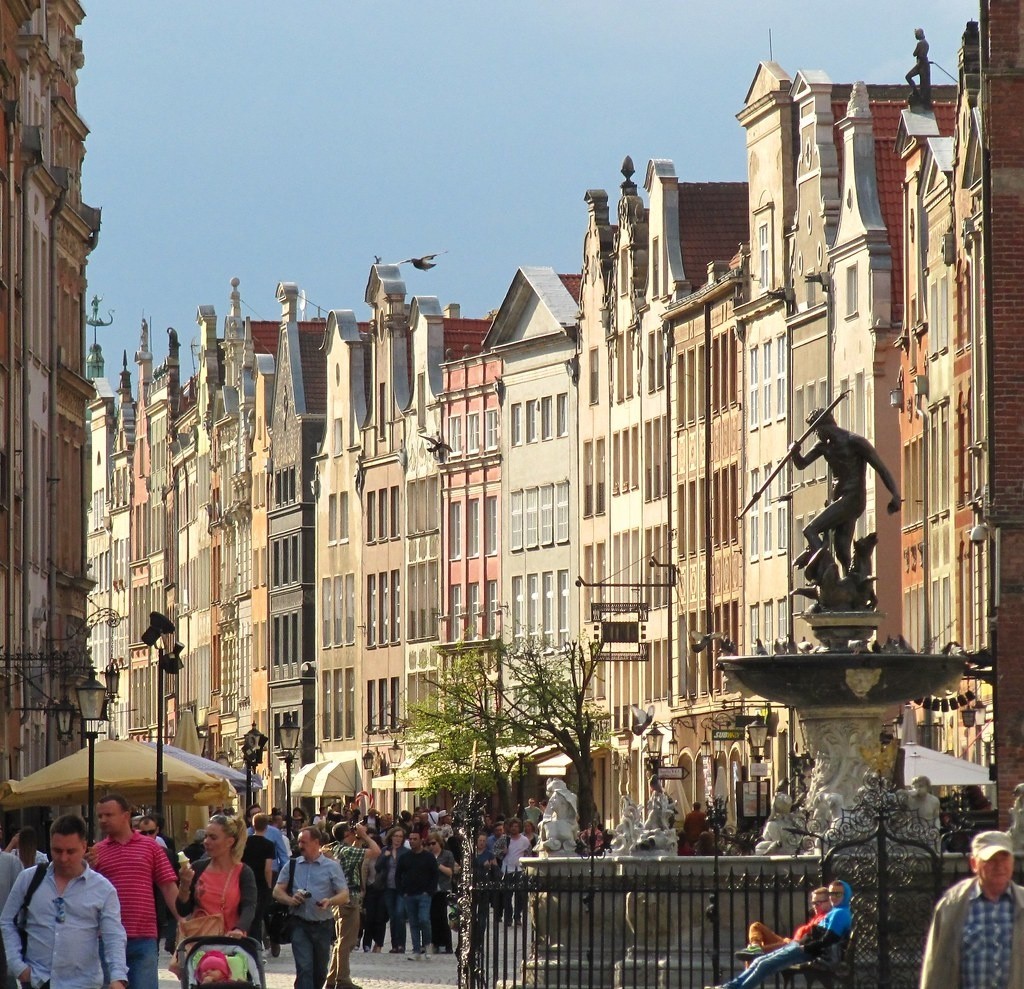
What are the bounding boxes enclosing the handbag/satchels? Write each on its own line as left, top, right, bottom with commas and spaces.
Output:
268, 914, 291, 945
169, 912, 224, 978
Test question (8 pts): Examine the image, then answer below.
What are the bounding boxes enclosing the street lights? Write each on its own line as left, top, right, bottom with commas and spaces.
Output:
141, 611, 185, 831
747, 715, 769, 826
278, 712, 300, 840
516, 736, 526, 819
388, 739, 404, 826
241, 721, 262, 828
75, 669, 108, 847
645, 721, 664, 775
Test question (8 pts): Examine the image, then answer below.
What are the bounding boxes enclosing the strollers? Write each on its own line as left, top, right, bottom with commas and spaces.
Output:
176, 934, 266, 989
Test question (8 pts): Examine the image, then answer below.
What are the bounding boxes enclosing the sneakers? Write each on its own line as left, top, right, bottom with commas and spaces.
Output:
735, 945, 764, 961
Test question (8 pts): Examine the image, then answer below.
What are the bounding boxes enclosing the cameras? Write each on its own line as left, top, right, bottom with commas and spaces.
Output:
352, 820, 365, 830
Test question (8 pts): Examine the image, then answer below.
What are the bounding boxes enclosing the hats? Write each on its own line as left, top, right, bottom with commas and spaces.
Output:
971, 831, 1013, 861
438, 810, 449, 818
200, 956, 228, 977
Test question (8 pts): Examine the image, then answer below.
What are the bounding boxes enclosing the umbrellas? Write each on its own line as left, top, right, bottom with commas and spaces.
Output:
902, 740, 997, 786
162, 709, 209, 848
0, 737, 262, 809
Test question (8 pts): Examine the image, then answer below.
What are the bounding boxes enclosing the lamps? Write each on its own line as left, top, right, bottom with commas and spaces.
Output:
20, 646, 75, 744
300, 661, 316, 675
85, 294, 115, 380
46, 608, 121, 704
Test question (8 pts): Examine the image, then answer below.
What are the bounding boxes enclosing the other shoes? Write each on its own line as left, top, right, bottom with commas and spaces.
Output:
515, 919, 521, 926
354, 945, 454, 961
337, 982, 362, 989
705, 984, 724, 989
326, 984, 335, 989
506, 918, 512, 926
270, 941, 281, 958
165, 940, 176, 955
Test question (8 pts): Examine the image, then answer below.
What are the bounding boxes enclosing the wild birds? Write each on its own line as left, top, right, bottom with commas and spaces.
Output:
773, 634, 797, 655
628, 705, 654, 736
755, 638, 769, 655
417, 434, 453, 453
394, 250, 449, 271
872, 634, 916, 655
797, 636, 830, 655
847, 639, 871, 654
939, 641, 968, 657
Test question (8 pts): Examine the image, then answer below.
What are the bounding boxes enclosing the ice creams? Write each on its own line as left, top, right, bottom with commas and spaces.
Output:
178, 851, 190, 868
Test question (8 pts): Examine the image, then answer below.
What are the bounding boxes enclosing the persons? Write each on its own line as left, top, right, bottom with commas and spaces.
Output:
704, 880, 851, 989
212, 798, 547, 989
0, 814, 129, 989
131, 811, 207, 952
200, 957, 246, 984
141, 318, 148, 346
678, 802, 716, 855
905, 28, 931, 101
921, 832, 1024, 989
175, 816, 257, 939
790, 408, 902, 586
0, 826, 49, 989
900, 775, 947, 853
84, 796, 186, 989
91, 294, 103, 320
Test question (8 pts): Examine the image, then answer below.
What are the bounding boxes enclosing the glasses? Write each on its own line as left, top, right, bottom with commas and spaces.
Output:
829, 892, 844, 897
53, 898, 66, 923
139, 827, 158, 836
208, 815, 227, 825
811, 900, 827, 906
427, 840, 437, 847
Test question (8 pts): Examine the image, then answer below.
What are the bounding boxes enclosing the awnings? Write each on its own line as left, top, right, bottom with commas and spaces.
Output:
371, 743, 563, 789
535, 747, 601, 775
291, 760, 356, 797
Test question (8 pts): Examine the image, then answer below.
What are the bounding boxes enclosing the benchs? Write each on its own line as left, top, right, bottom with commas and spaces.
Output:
758, 925, 858, 989
